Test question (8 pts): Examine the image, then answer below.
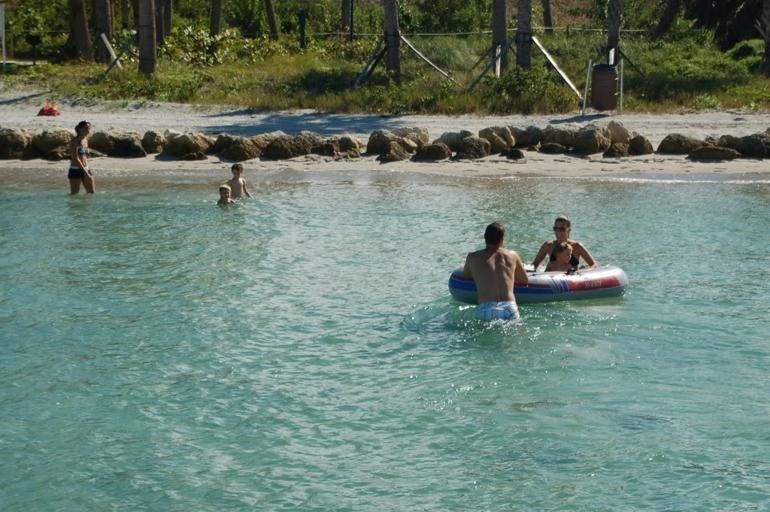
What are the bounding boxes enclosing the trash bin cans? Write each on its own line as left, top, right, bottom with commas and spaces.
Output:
592, 63, 618, 110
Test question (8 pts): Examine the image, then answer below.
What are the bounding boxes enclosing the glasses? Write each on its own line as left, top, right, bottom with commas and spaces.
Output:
553, 227, 566, 231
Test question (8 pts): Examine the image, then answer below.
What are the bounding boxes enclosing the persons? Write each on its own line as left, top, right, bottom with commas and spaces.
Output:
218, 164, 254, 207
68, 121, 95, 195
463, 223, 528, 324
532, 216, 597, 273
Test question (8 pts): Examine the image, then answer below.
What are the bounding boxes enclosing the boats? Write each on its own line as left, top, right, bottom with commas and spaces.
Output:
447, 261, 628, 305
